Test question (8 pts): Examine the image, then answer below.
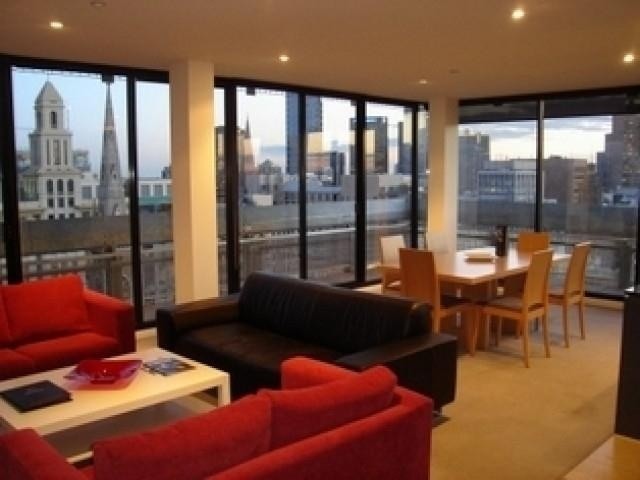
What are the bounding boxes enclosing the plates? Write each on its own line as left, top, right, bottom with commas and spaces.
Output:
464, 252, 501, 261
63, 358, 144, 387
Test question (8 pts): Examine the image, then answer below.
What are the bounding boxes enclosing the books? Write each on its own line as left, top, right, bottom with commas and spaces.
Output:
1, 380, 70, 413
143, 357, 194, 376
464, 249, 500, 263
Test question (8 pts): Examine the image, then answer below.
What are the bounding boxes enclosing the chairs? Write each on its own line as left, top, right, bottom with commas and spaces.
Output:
372, 227, 593, 371
